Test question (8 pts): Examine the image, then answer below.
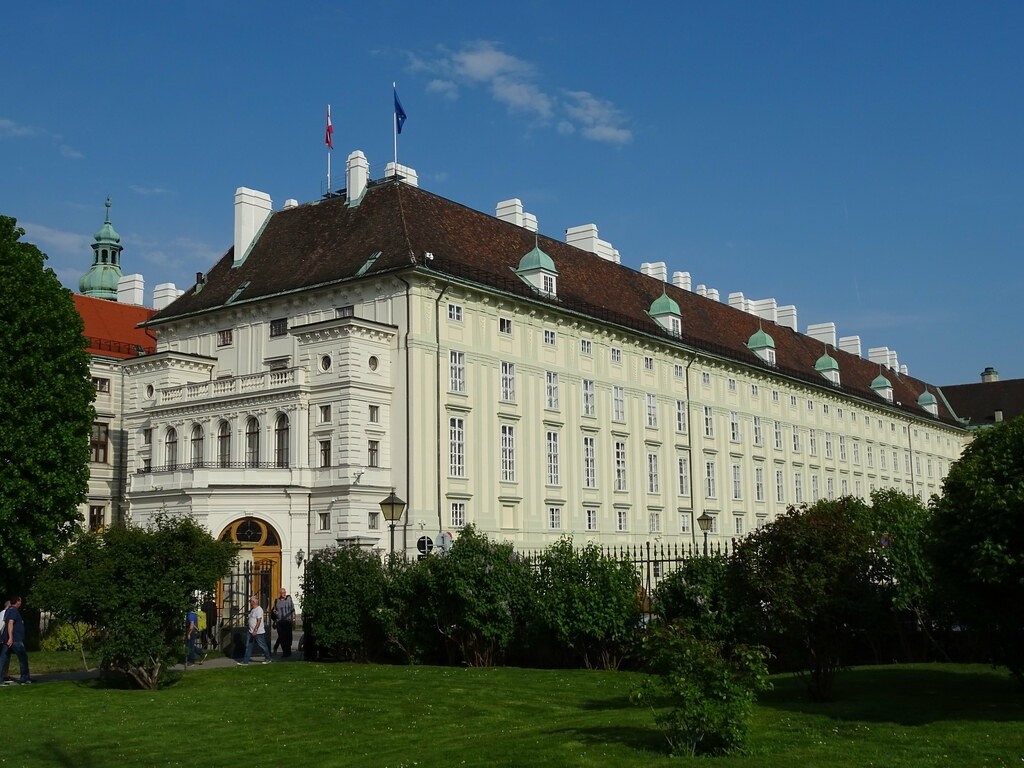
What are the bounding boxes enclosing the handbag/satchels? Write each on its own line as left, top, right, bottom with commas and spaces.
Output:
270, 611, 277, 621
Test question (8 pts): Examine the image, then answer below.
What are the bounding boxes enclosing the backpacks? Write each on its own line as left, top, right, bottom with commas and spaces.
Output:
190, 611, 208, 632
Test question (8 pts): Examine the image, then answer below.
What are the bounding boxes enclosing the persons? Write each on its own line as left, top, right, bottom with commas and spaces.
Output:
237, 596, 271, 666
270, 588, 296, 657
184, 595, 217, 666
0, 596, 37, 686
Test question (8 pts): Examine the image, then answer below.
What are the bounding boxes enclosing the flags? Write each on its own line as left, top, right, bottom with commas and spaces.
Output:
393, 87, 407, 134
325, 105, 333, 150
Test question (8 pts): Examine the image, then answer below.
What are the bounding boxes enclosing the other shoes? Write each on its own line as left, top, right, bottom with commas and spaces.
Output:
0, 680, 14, 686
213, 642, 218, 649
237, 662, 248, 666
188, 662, 194, 666
200, 653, 207, 664
22, 680, 33, 685
263, 660, 271, 664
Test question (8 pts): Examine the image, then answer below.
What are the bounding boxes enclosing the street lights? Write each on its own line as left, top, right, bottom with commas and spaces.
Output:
697, 509, 714, 561
379, 488, 407, 561
